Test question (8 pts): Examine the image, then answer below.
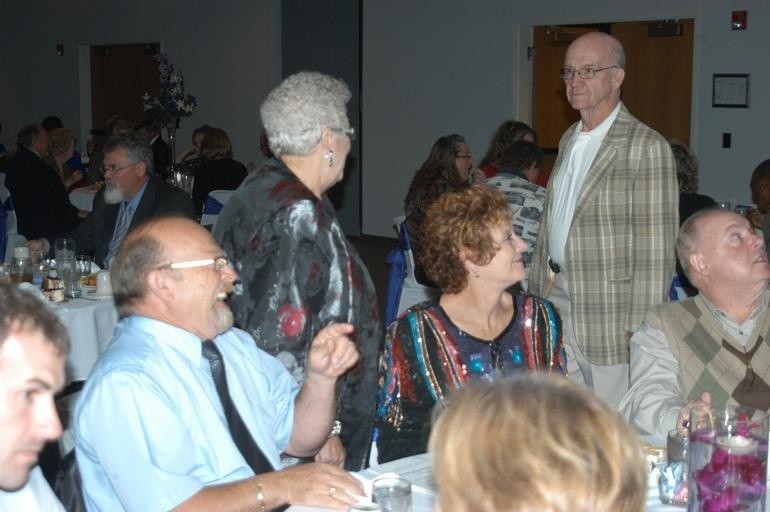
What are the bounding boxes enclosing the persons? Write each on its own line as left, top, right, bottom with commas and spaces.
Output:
2, 29, 768, 512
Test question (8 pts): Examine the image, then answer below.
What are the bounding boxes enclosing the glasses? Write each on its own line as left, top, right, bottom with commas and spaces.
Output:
157, 257, 229, 273
558, 65, 619, 80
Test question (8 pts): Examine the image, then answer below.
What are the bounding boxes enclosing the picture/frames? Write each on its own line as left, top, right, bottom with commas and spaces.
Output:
711, 72, 749, 109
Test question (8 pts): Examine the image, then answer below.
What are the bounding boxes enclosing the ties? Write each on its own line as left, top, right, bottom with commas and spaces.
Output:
106, 204, 131, 261
201, 339, 290, 509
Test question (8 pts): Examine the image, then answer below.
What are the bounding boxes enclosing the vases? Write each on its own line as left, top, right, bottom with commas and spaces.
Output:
159, 117, 176, 175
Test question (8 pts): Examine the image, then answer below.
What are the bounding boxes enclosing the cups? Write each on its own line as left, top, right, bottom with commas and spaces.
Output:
372, 475, 412, 511
169, 167, 194, 197
8, 237, 112, 301
659, 402, 767, 511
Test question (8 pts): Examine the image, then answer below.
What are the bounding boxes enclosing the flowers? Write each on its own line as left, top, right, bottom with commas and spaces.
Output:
140, 50, 199, 131
